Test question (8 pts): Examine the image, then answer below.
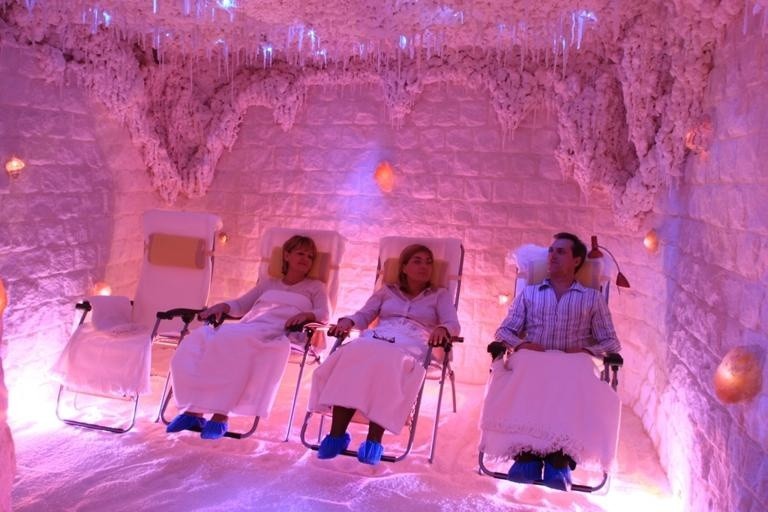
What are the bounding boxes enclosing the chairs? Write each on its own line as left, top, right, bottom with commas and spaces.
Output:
478, 243, 626, 496
157, 228, 349, 444
51, 208, 229, 437
297, 234, 466, 465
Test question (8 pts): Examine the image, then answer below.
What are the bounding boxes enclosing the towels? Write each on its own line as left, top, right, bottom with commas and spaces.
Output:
68, 289, 149, 339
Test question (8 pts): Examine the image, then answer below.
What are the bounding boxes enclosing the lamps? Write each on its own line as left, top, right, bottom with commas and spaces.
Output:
583, 233, 632, 294
5, 152, 27, 181
710, 342, 761, 407
642, 228, 660, 254
374, 157, 396, 194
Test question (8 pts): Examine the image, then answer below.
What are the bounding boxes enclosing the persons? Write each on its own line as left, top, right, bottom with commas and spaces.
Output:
168, 235, 332, 438
496, 231, 620, 492
317, 244, 462, 463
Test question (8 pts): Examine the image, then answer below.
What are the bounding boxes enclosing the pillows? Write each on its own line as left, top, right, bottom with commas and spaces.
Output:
381, 255, 449, 291
143, 231, 208, 272
268, 245, 332, 284
523, 255, 604, 291
